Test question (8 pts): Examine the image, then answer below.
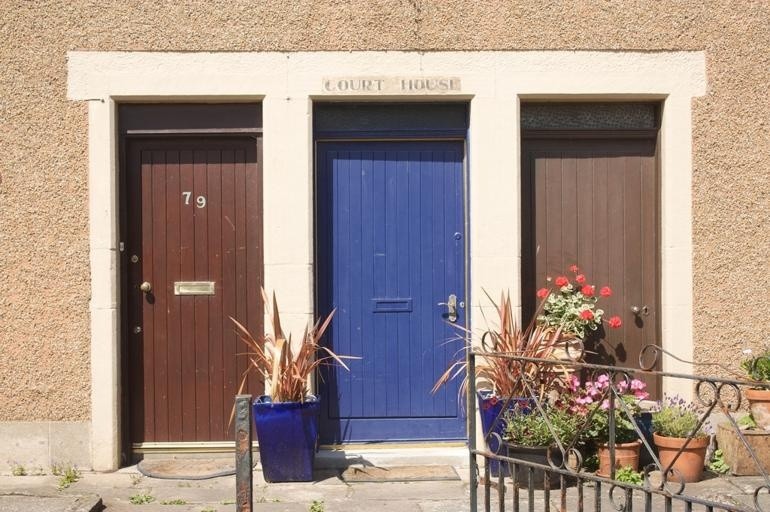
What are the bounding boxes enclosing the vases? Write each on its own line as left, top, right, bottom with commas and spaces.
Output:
501, 441, 575, 493
597, 439, 646, 480
653, 431, 711, 484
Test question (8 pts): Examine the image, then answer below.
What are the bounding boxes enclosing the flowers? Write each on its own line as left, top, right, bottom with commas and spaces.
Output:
537, 262, 622, 336
480, 394, 595, 450
649, 391, 715, 440
569, 373, 649, 439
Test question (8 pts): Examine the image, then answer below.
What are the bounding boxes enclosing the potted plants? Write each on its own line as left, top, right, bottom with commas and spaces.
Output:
741, 351, 770, 430
